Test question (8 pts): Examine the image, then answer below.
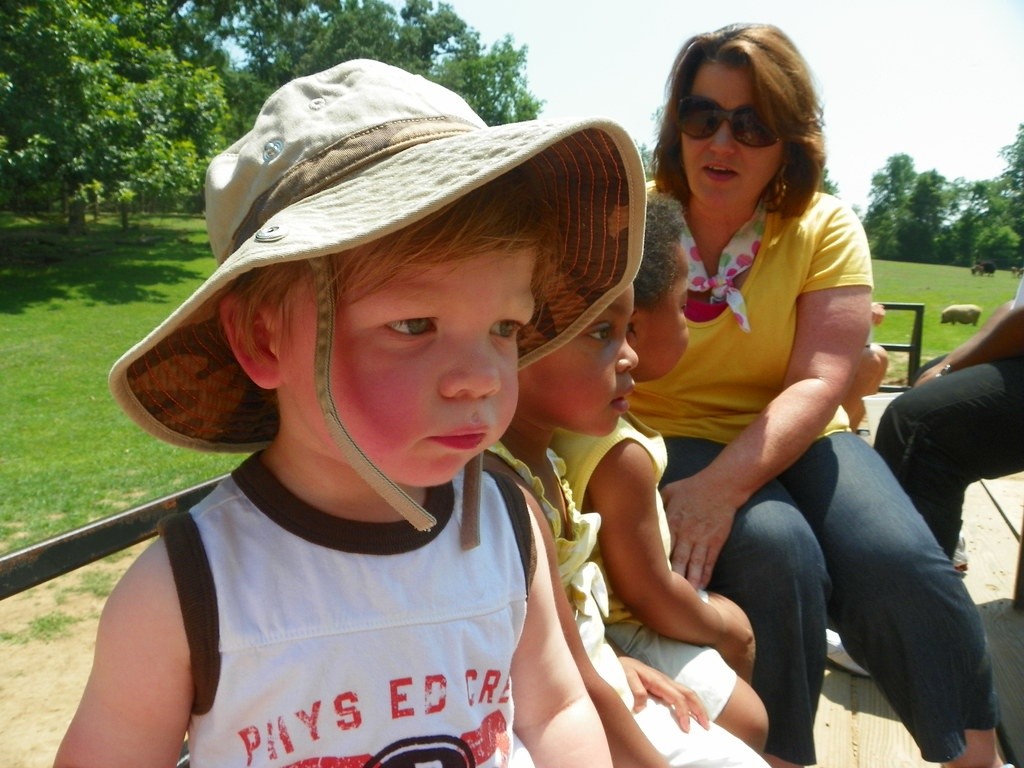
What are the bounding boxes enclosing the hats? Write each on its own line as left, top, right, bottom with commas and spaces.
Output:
106, 57, 650, 551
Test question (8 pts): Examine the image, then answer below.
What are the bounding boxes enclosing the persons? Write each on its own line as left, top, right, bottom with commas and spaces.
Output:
640, 21, 1020, 768
546, 191, 783, 768
495, 273, 777, 768
822, 275, 1024, 679
51, 52, 620, 768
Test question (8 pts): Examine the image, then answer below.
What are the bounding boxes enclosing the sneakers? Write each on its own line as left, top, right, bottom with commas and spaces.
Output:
825, 629, 871, 679
953, 532, 968, 571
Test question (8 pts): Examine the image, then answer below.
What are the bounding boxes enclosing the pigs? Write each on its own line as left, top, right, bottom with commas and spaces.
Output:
940, 304, 982, 326
1011, 265, 1024, 278
971, 260, 996, 277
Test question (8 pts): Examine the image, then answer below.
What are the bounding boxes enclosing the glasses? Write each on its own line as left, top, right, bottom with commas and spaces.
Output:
675, 94, 780, 149
